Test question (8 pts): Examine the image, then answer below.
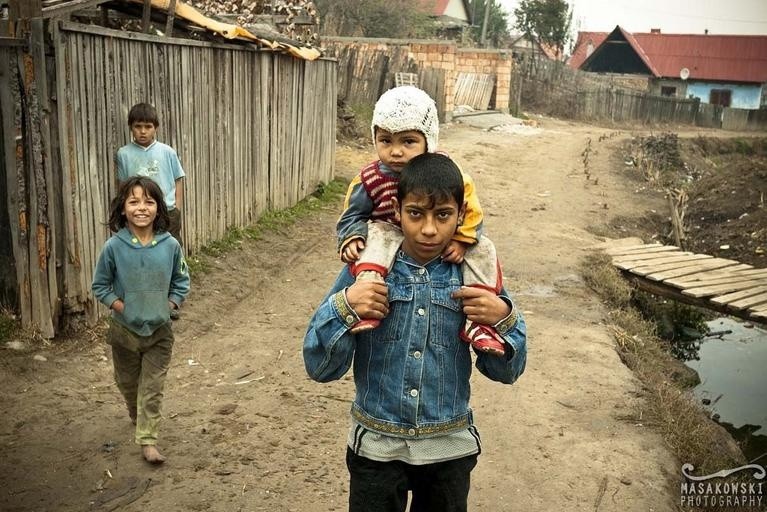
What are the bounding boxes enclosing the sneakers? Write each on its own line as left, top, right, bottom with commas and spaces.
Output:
460, 319, 505, 356
349, 319, 381, 334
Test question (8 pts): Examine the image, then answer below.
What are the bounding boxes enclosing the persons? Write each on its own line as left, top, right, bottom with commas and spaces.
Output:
92, 176, 190, 463
303, 153, 526, 512
116, 103, 186, 320
336, 86, 506, 357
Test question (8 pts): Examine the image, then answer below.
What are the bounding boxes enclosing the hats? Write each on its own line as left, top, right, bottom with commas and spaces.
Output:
371, 85, 439, 153
